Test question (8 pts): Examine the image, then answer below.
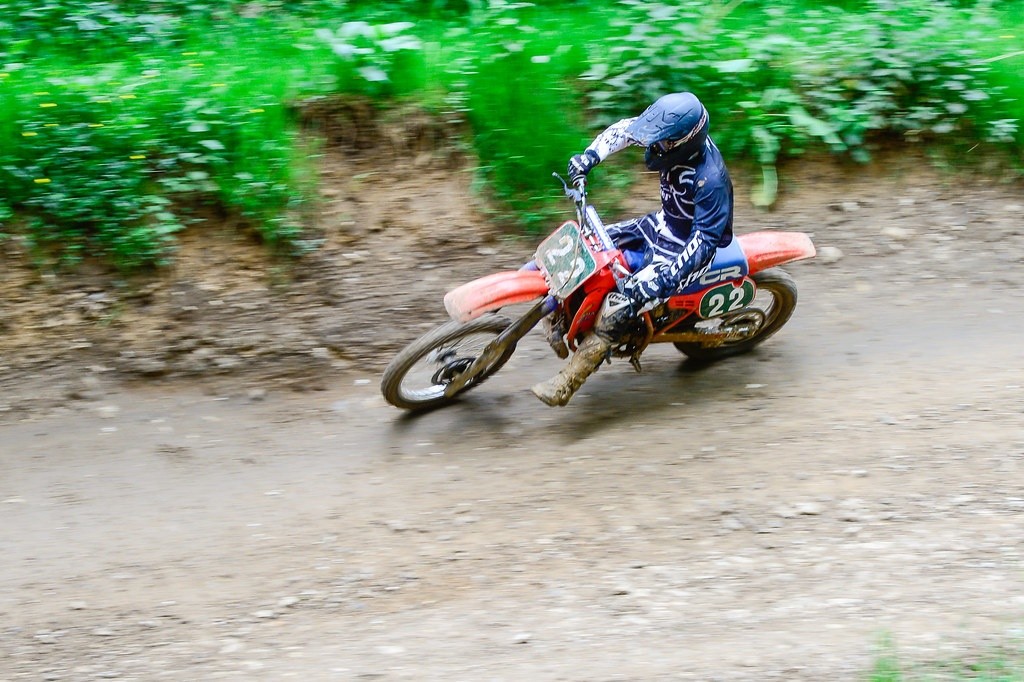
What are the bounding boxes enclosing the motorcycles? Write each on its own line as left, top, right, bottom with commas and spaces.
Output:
381, 171, 816, 410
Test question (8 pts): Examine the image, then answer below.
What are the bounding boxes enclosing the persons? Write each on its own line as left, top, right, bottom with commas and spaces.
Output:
531, 93, 734, 409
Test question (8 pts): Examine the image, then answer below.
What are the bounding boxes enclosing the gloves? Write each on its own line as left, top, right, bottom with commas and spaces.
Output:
632, 274, 673, 306
567, 150, 601, 181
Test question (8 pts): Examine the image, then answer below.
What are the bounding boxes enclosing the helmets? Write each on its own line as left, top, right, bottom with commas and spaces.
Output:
626, 92, 709, 171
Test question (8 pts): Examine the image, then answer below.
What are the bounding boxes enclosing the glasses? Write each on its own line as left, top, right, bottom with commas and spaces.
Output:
649, 139, 674, 154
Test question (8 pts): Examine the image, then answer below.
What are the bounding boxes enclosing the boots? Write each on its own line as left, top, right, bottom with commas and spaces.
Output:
530, 332, 609, 407
